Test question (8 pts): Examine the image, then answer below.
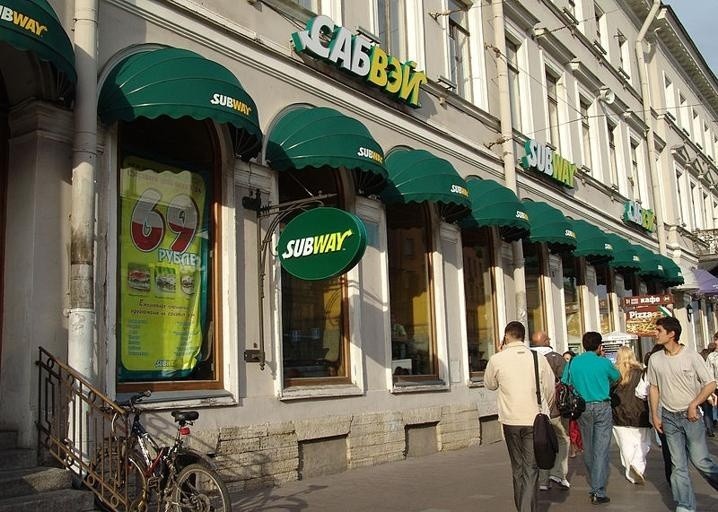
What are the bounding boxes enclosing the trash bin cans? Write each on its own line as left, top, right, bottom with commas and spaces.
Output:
153, 447, 196, 503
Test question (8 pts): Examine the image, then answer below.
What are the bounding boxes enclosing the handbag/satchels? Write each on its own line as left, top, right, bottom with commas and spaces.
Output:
555, 353, 587, 422
528, 348, 559, 471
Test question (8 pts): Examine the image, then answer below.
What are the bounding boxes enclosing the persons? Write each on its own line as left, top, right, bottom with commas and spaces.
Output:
391, 312, 409, 359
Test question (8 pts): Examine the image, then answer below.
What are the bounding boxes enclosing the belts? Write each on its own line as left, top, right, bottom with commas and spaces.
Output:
583, 397, 613, 405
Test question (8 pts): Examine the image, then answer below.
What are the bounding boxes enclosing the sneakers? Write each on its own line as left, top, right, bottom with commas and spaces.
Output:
548, 473, 571, 489
538, 481, 554, 491
629, 463, 646, 484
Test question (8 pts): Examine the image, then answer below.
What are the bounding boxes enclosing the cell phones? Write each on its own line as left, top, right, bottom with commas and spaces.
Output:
500, 335, 505, 349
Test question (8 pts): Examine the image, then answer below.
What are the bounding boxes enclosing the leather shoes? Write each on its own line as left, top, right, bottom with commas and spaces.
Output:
589, 491, 611, 506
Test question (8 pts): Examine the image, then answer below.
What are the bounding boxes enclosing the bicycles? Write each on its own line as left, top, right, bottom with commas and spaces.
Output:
74, 388, 234, 511
